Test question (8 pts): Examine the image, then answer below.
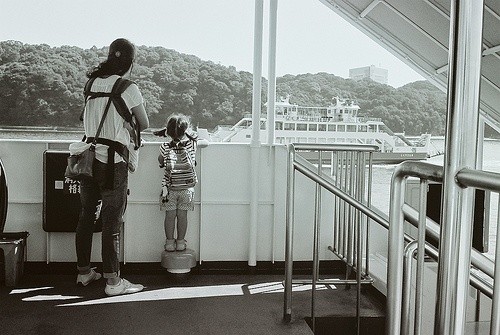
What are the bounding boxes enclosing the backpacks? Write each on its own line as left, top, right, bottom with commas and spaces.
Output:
166, 142, 198, 191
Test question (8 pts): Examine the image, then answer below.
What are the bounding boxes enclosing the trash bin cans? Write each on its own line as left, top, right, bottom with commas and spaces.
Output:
0, 231, 30, 287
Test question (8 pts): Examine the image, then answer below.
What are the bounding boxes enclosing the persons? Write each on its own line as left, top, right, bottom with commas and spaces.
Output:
152, 113, 210, 252
75, 39, 150, 297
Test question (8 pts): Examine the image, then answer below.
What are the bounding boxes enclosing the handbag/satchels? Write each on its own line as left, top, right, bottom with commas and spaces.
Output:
64, 141, 93, 178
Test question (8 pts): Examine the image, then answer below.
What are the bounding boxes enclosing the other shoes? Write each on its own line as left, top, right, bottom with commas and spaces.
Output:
76, 267, 102, 287
105, 278, 143, 296
176, 239, 187, 251
165, 238, 178, 252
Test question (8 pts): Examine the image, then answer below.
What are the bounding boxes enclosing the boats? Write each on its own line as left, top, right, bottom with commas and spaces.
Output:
197, 93, 445, 165
